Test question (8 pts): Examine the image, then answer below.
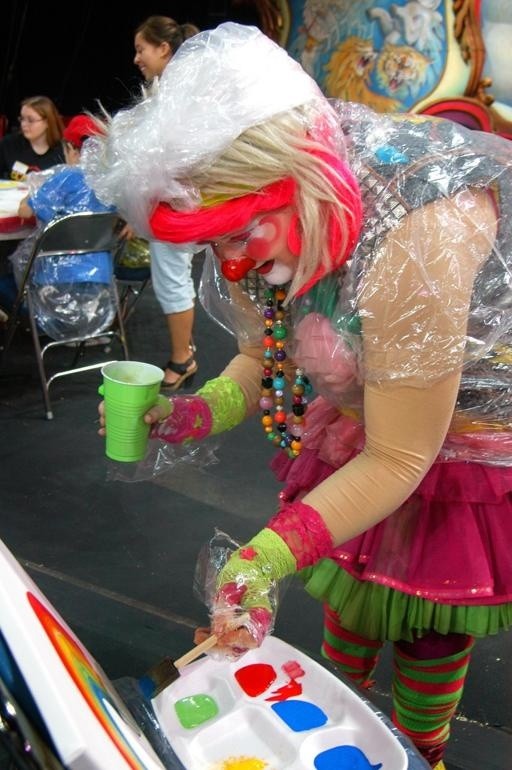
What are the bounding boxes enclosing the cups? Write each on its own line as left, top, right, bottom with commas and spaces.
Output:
100, 360, 167, 464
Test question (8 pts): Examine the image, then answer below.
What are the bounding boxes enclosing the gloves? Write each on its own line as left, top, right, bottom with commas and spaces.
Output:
211, 495, 346, 664
149, 373, 249, 452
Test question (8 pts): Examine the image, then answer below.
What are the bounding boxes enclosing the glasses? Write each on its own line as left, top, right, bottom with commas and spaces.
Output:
17, 115, 47, 124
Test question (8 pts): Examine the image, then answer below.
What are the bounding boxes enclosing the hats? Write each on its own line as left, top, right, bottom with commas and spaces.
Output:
62, 111, 106, 151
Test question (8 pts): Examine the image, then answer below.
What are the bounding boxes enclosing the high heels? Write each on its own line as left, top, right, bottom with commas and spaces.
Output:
159, 353, 199, 394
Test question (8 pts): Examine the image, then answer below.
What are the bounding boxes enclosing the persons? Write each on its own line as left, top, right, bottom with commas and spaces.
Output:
16, 114, 126, 350
97, 23, 512, 770
93, 18, 199, 391
10, 91, 73, 182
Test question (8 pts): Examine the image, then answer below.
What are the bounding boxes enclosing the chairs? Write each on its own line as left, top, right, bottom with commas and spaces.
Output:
2, 210, 129, 422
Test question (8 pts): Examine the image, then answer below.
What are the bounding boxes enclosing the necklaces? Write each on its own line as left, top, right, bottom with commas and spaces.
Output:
258, 287, 313, 459
